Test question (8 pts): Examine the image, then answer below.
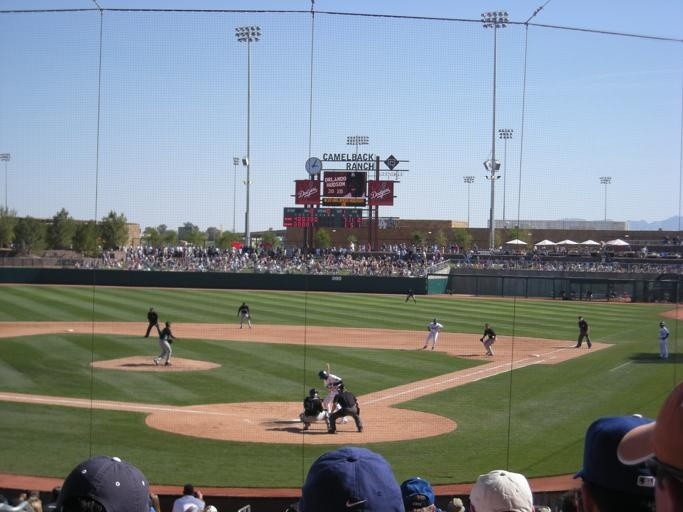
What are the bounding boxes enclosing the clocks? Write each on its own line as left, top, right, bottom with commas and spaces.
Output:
304, 157, 321, 175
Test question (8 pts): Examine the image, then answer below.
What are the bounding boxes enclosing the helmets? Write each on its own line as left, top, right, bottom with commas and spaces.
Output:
318, 370, 327, 376
310, 388, 318, 395
338, 383, 345, 390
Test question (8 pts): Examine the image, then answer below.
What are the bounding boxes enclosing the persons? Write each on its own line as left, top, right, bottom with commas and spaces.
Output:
571, 412, 655, 509
142, 307, 162, 338
237, 302, 252, 329
658, 320, 669, 359
152, 321, 175, 366
74, 240, 681, 304
297, 362, 363, 435
617, 383, 682, 510
1, 468, 605, 512
423, 317, 443, 351
479, 323, 497, 355
574, 315, 593, 348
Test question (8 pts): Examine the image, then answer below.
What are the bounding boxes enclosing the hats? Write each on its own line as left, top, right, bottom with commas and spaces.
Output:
400, 477, 435, 509
572, 413, 656, 488
617, 382, 683, 471
448, 498, 465, 512
469, 470, 533, 512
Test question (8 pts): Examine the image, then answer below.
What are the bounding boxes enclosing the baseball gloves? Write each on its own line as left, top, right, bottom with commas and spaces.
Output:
479, 338, 483, 342
168, 339, 174, 343
247, 316, 250, 319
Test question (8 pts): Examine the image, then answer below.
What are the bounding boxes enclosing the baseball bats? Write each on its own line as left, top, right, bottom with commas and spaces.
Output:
324, 362, 333, 389
